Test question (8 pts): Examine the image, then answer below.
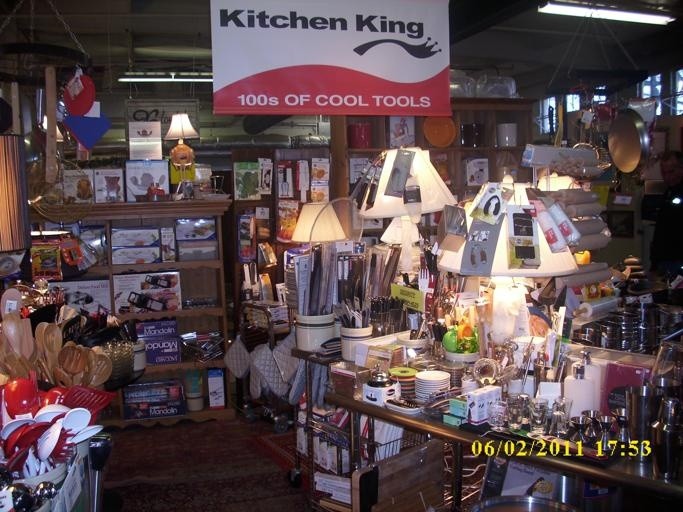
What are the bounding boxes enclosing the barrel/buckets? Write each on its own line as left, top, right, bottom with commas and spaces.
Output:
294, 313, 334, 351
574, 312, 647, 354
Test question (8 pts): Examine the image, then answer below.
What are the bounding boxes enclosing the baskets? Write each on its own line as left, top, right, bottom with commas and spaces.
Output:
259, 430, 340, 499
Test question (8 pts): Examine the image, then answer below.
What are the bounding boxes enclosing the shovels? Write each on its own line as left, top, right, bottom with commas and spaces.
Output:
1, 287, 117, 511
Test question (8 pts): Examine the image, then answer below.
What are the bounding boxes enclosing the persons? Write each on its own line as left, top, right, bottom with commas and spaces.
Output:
649, 149, 682, 283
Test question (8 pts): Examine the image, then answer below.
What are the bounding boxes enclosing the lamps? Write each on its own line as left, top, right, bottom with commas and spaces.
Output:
118, 75, 213, 84
536, 0, 676, 26
292, 201, 347, 243
360, 146, 458, 271
164, 112, 200, 162
437, 201, 579, 339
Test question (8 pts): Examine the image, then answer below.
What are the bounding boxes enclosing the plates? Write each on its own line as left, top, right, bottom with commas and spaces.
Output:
414, 370, 451, 406
607, 107, 651, 176
385, 395, 425, 416
202, 193, 232, 201
421, 112, 457, 148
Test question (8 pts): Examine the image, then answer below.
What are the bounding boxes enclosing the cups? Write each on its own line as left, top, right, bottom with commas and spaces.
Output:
444, 350, 481, 369
488, 394, 631, 454
461, 122, 481, 148
9, 462, 68, 494
186, 391, 204, 412
623, 375, 682, 487
294, 311, 335, 355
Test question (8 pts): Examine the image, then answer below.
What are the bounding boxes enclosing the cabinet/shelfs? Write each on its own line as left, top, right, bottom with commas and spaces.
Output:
287, 349, 376, 510
29, 196, 234, 423
327, 339, 681, 511
232, 143, 332, 422
331, 102, 459, 302
459, 99, 541, 202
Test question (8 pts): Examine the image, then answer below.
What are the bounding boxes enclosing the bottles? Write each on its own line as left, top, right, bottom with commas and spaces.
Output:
525, 199, 568, 253
348, 120, 373, 149
542, 196, 583, 246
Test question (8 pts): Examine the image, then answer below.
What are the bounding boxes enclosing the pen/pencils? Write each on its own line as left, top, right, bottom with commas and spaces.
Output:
338, 295, 403, 338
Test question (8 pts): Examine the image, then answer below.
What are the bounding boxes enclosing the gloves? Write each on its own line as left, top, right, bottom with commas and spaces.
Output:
249, 344, 331, 410
